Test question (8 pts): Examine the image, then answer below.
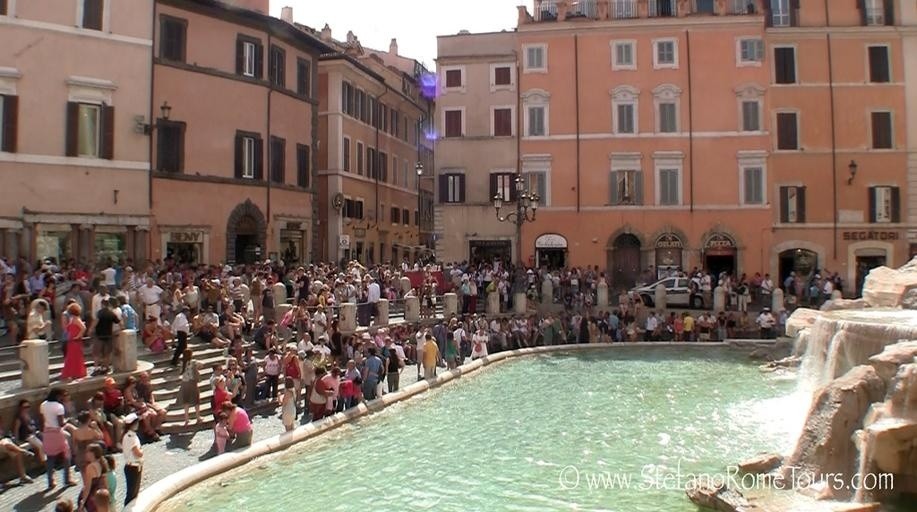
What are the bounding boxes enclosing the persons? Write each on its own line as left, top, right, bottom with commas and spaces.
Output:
0, 238, 884, 511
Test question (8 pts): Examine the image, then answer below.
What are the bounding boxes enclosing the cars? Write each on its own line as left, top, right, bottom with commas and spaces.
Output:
630, 277, 706, 310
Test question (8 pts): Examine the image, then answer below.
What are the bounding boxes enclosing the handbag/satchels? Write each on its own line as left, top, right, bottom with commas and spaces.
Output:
192, 367, 200, 383
310, 390, 326, 404
78, 488, 96, 512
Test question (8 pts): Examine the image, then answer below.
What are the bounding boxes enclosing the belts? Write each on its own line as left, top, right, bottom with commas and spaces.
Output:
127, 462, 141, 467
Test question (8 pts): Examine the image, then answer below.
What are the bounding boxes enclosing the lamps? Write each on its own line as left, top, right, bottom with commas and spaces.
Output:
847, 160, 857, 184
415, 161, 423, 177
160, 102, 171, 119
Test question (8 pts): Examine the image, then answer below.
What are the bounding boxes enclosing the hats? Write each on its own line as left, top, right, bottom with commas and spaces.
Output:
125, 412, 141, 424
105, 378, 115, 389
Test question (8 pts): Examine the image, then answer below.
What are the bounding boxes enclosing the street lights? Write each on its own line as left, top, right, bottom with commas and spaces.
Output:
493, 175, 541, 269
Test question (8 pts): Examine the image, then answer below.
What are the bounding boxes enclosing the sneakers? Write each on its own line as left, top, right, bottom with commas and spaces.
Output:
21, 476, 34, 482
66, 481, 77, 486
91, 367, 111, 376
49, 480, 56, 489
144, 347, 152, 352
23, 450, 34, 457
156, 430, 164, 436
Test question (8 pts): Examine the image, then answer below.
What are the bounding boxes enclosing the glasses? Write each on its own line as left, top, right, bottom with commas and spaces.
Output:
23, 406, 32, 409
